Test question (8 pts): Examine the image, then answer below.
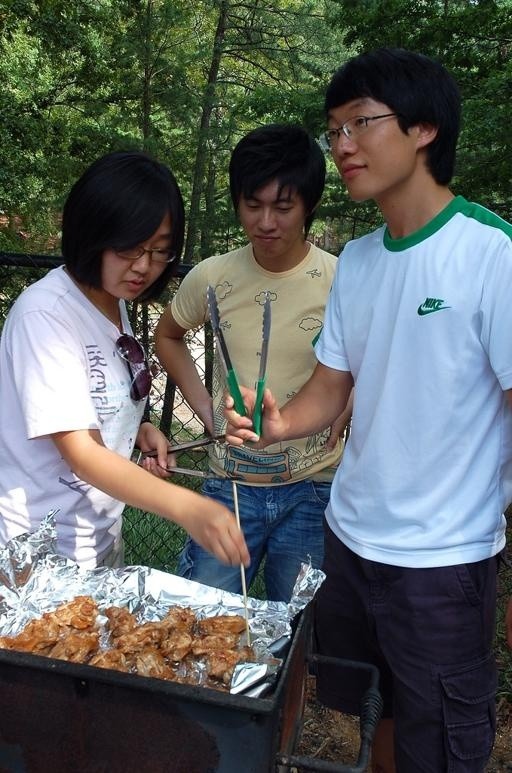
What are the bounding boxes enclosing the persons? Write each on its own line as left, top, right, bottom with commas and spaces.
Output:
0, 143, 250, 610
154, 121, 352, 605
229, 47, 511, 772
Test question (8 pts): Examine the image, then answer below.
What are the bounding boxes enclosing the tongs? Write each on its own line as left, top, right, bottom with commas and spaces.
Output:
206, 284, 274, 441
145, 435, 248, 483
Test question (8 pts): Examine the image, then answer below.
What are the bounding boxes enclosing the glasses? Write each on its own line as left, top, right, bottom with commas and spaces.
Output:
315, 114, 395, 156
113, 246, 178, 265
114, 333, 153, 402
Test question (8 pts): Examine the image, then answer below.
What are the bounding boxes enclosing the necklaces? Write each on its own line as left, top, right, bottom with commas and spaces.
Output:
81, 286, 120, 327
